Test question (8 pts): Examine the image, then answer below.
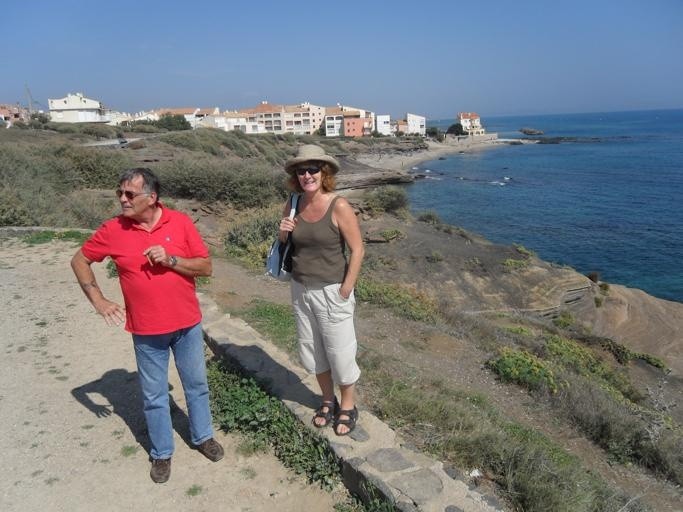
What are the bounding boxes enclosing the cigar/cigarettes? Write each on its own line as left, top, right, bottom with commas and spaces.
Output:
144, 249, 153, 267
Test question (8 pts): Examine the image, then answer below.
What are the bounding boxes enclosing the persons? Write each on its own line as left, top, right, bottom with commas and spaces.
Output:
267, 144, 364, 434
68, 168, 224, 483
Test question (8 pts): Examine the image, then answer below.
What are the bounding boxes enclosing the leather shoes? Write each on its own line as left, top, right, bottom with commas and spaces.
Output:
151, 458, 172, 483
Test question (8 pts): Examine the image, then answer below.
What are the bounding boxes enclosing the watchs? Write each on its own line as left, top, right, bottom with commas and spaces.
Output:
167, 253, 176, 272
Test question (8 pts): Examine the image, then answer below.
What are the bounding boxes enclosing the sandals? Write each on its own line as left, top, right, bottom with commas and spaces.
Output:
312, 396, 339, 428
334, 405, 358, 436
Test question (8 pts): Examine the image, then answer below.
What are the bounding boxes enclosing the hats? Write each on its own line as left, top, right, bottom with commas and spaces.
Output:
284, 144, 340, 176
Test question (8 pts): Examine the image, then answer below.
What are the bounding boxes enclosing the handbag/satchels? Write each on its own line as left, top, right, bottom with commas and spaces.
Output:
198, 437, 224, 462
267, 193, 299, 281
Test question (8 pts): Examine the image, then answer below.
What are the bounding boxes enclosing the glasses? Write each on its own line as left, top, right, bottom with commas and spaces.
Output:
116, 190, 150, 199
297, 167, 319, 175
